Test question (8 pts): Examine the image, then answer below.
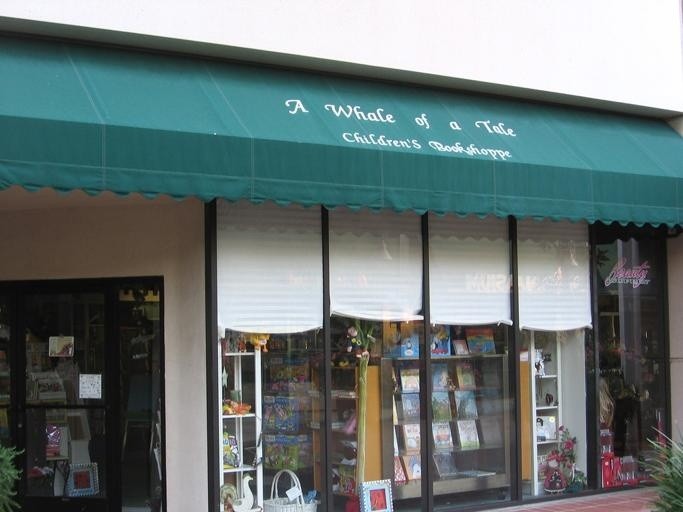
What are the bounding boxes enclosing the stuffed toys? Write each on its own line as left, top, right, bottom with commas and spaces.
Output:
345, 323, 373, 357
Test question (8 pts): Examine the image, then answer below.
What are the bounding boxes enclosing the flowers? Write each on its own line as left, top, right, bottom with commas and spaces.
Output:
345, 319, 382, 357
545, 425, 578, 469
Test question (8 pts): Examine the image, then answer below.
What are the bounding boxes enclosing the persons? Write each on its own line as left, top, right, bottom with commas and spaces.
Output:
535, 416, 547, 436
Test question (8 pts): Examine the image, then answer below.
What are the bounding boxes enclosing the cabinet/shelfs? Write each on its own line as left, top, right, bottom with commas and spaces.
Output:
310, 353, 510, 504
218, 332, 264, 512
521, 329, 563, 496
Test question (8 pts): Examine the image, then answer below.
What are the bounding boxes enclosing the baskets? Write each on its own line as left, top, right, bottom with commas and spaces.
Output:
263, 469, 320, 512
